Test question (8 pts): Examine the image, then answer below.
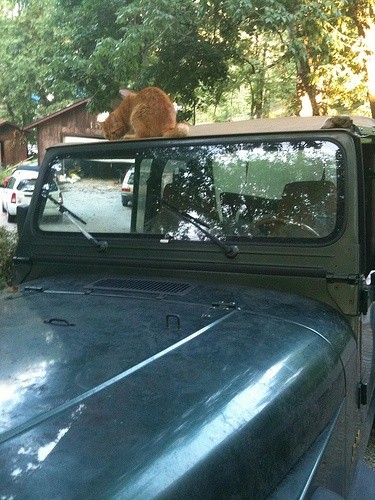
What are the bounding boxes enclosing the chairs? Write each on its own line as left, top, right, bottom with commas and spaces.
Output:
270, 181, 336, 237
150, 183, 213, 234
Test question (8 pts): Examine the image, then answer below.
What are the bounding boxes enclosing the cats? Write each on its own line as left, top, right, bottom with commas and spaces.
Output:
98, 86, 189, 140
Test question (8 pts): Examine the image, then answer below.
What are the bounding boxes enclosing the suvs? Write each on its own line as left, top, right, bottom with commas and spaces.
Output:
0, 166, 64, 224
1, 111, 374, 499
120, 164, 164, 207
